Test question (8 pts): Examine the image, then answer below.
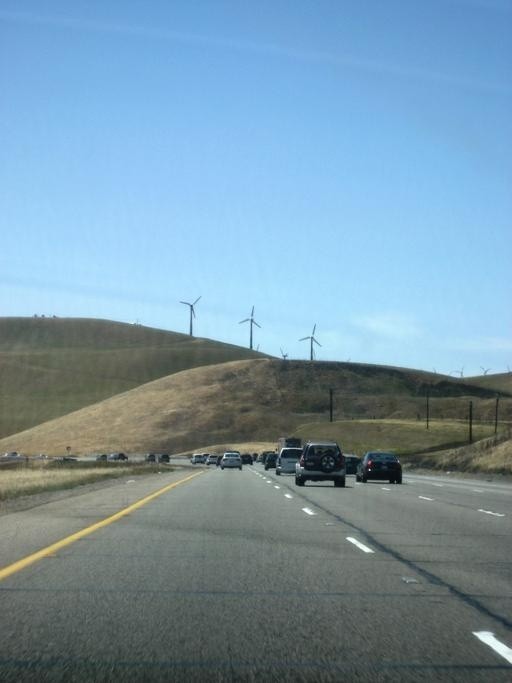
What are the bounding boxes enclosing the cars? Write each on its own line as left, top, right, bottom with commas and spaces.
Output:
188, 435, 402, 488
4, 451, 170, 464
